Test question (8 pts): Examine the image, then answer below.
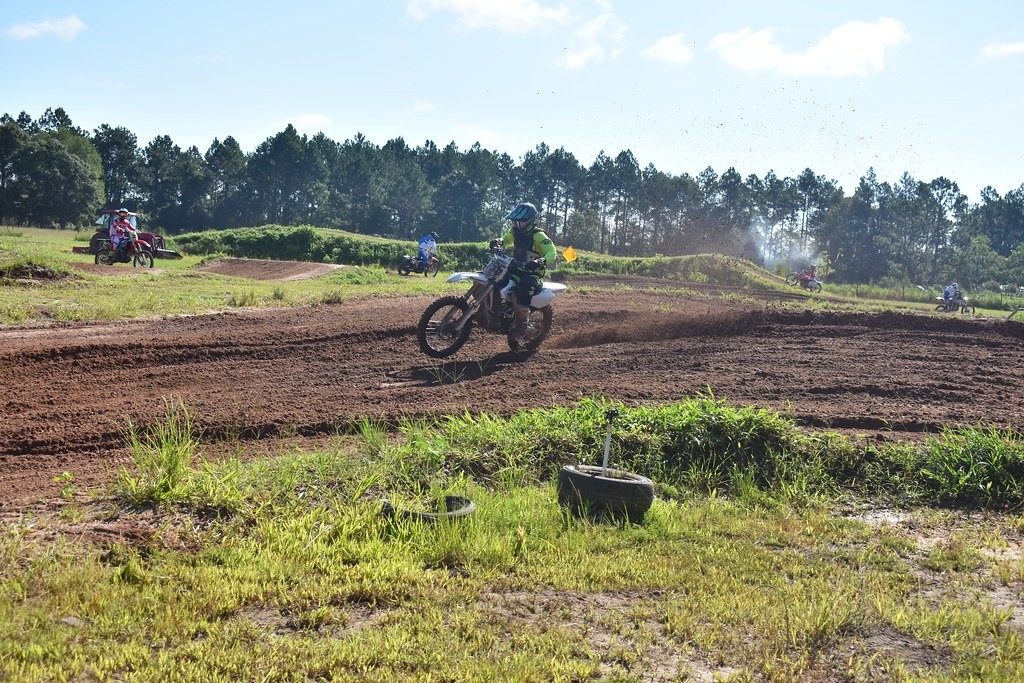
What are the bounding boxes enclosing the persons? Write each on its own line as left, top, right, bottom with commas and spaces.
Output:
109, 208, 141, 258
417, 232, 440, 266
489, 202, 557, 341
801, 264, 816, 286
944, 283, 959, 311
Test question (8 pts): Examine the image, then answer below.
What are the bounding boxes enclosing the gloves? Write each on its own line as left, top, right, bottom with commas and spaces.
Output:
135, 229, 141, 234
490, 238, 503, 250
530, 257, 546, 271
116, 226, 123, 231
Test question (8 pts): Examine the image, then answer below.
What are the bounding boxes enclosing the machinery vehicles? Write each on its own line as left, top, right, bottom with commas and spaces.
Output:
72, 209, 183, 261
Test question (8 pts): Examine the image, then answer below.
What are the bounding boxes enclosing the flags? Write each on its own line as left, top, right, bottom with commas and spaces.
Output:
563, 248, 576, 263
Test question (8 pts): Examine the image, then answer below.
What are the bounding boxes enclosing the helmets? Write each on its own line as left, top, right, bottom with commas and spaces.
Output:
506, 203, 539, 236
952, 283, 958, 289
810, 265, 816, 270
118, 208, 128, 218
429, 231, 439, 240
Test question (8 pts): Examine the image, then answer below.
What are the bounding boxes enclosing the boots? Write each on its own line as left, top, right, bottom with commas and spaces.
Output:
107, 251, 115, 265
510, 305, 530, 336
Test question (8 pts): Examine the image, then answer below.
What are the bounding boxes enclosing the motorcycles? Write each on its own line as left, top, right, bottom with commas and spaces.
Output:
95, 231, 154, 268
786, 270, 823, 294
417, 243, 567, 359
934, 292, 975, 315
398, 252, 439, 277
